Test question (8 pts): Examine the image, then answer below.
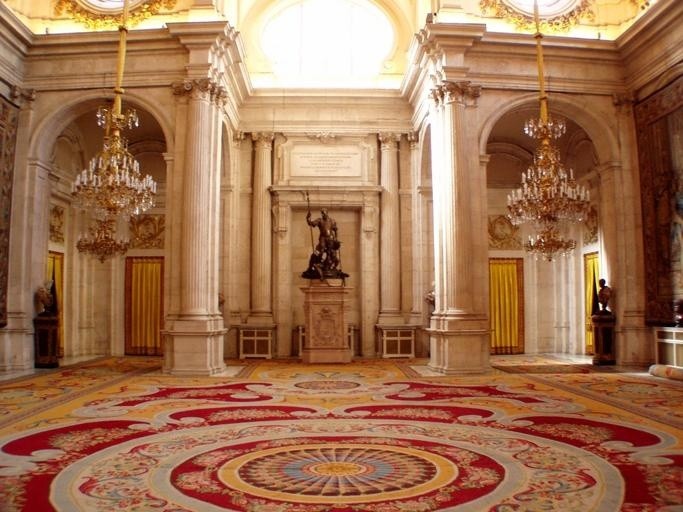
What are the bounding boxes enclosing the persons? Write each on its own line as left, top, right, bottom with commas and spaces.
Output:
596, 278, 611, 307
305, 207, 342, 282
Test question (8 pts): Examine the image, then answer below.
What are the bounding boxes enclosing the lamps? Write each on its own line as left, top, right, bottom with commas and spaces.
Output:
504, 0, 590, 263
67, 1, 157, 264
